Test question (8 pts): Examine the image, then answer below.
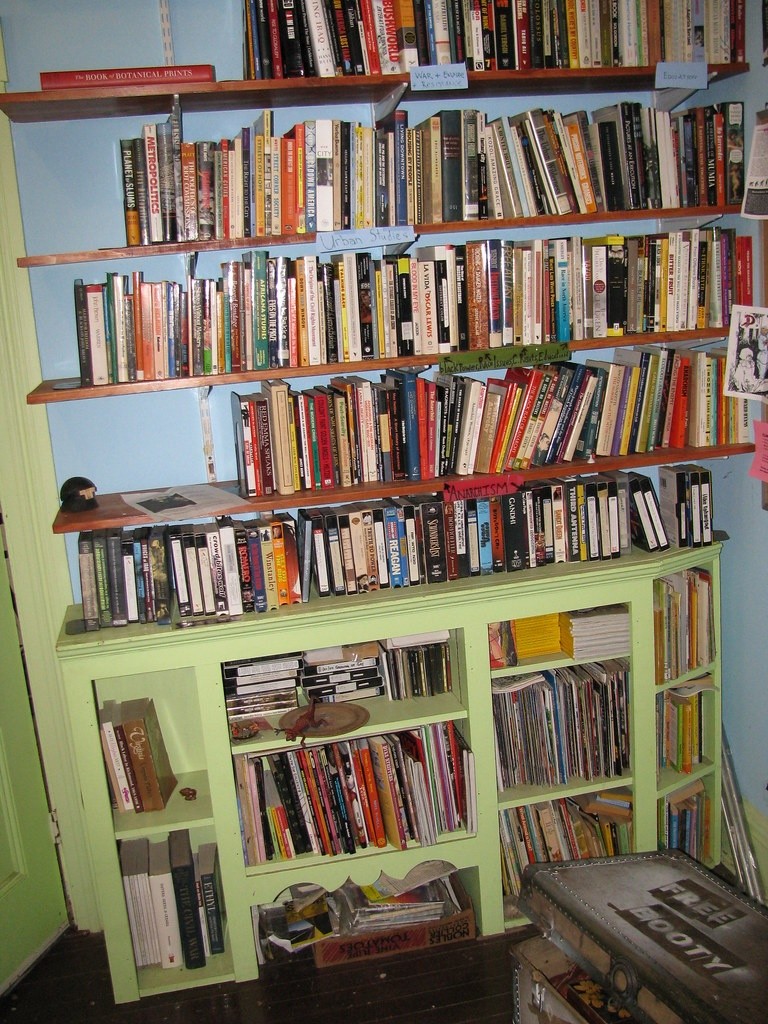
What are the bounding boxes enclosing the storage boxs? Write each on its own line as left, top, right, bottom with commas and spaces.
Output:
310, 870, 479, 968
507, 846, 767, 1024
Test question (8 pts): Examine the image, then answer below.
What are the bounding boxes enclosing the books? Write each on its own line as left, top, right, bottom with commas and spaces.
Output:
249, 861, 463, 964
120, 102, 744, 247
656, 691, 703, 783
79, 465, 713, 631
39, 65, 213, 90
74, 226, 752, 387
233, 720, 477, 866
221, 629, 452, 723
118, 829, 227, 969
488, 604, 630, 670
98, 698, 178, 813
507, 848, 768, 1024
657, 779, 711, 865
491, 658, 631, 791
242, 345, 750, 496
241, 0, 747, 81
498, 787, 635, 898
653, 566, 716, 685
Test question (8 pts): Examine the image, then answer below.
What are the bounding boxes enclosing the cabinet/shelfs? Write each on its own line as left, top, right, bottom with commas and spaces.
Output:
0, 1, 755, 1004
